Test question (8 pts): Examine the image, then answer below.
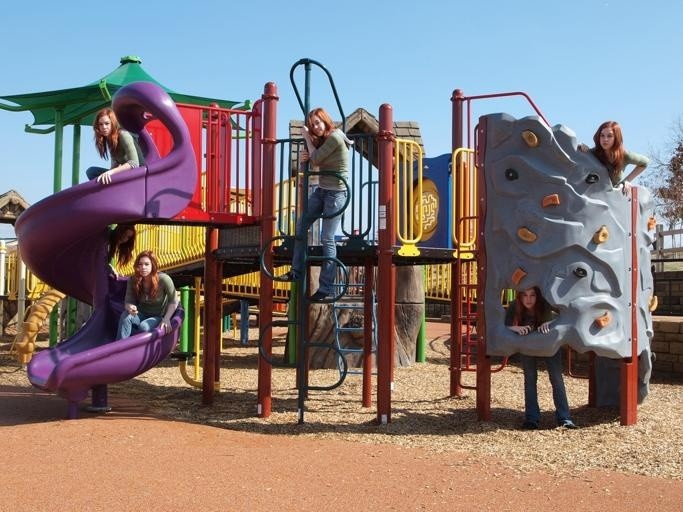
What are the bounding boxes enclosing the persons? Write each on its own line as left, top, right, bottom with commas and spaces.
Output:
116, 250, 178, 341
108, 223, 136, 282
504, 285, 579, 429
279, 107, 354, 302
86, 107, 145, 184
578, 121, 649, 196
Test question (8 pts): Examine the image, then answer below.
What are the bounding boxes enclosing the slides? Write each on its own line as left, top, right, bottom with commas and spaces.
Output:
14, 82, 197, 407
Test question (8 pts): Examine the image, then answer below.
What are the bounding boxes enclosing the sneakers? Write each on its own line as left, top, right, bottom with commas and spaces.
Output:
559, 418, 577, 429
519, 421, 538, 429
308, 291, 334, 303
275, 271, 298, 282
